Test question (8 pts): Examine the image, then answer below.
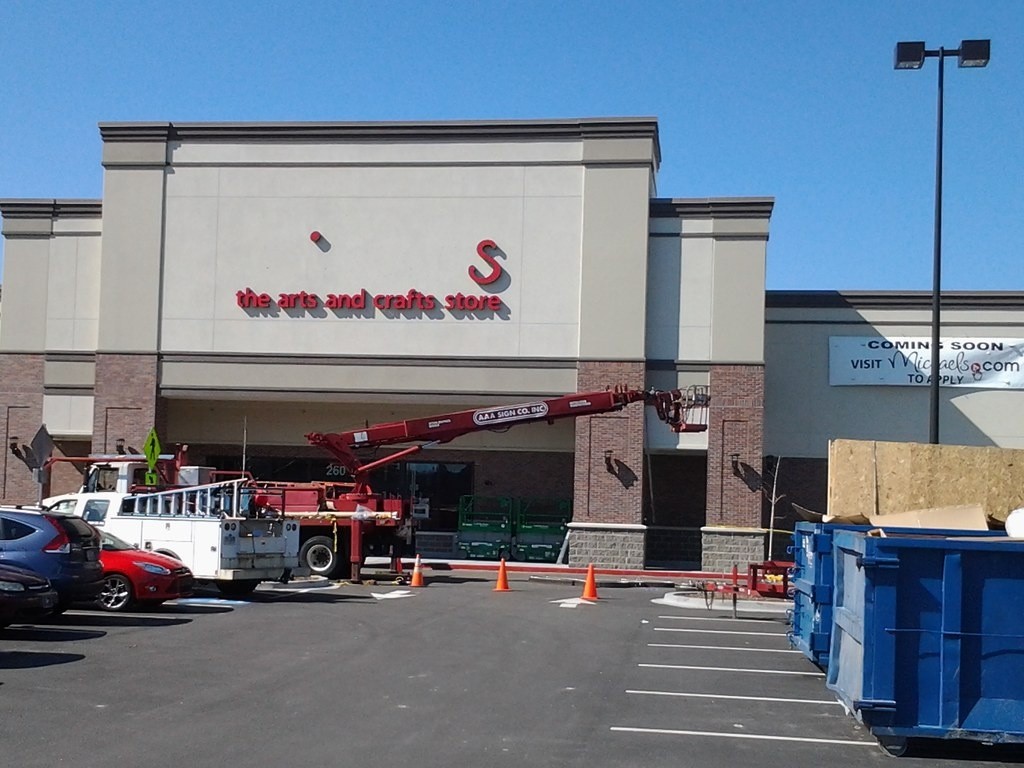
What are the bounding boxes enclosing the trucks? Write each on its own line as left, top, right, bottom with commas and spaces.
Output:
42, 491, 300, 600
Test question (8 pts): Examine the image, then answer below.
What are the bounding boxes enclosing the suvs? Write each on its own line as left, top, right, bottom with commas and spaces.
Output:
0, 503, 106, 630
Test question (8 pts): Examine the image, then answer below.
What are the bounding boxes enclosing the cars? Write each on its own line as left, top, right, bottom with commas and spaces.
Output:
94, 526, 197, 613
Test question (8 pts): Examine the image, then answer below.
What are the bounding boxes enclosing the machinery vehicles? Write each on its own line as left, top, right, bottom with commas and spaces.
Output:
36, 381, 712, 585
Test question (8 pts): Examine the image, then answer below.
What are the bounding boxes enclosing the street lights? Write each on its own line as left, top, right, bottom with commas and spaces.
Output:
892, 39, 990, 444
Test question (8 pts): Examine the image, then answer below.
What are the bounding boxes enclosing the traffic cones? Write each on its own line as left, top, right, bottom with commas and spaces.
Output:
579, 563, 601, 600
406, 553, 426, 588
492, 557, 513, 592
